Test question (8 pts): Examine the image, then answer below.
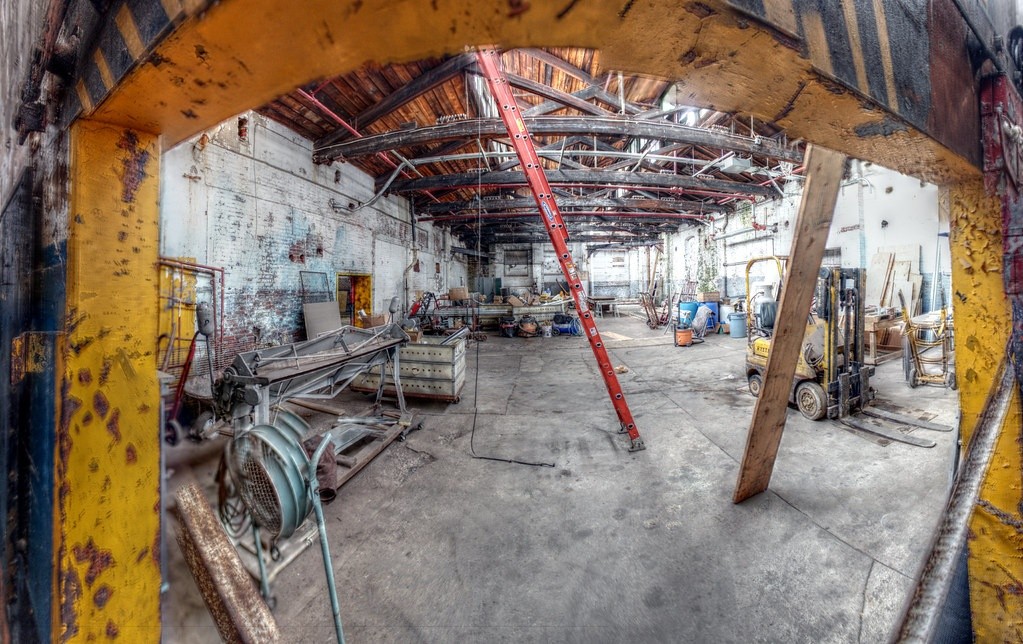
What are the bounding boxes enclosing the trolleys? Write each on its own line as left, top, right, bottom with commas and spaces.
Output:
897, 287, 958, 391
638, 290, 678, 329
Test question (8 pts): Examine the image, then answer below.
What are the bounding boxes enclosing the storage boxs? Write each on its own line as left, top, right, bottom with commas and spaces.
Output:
698, 291, 720, 302
450, 286, 470, 298
508, 295, 524, 306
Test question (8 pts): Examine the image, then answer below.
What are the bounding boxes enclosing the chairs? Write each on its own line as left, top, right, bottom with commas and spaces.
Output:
760, 301, 778, 336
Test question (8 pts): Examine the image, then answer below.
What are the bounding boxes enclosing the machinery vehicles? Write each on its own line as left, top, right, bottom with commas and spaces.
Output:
742, 256, 953, 449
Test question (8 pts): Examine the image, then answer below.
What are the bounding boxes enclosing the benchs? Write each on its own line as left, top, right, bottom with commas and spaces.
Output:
595, 301, 619, 319
